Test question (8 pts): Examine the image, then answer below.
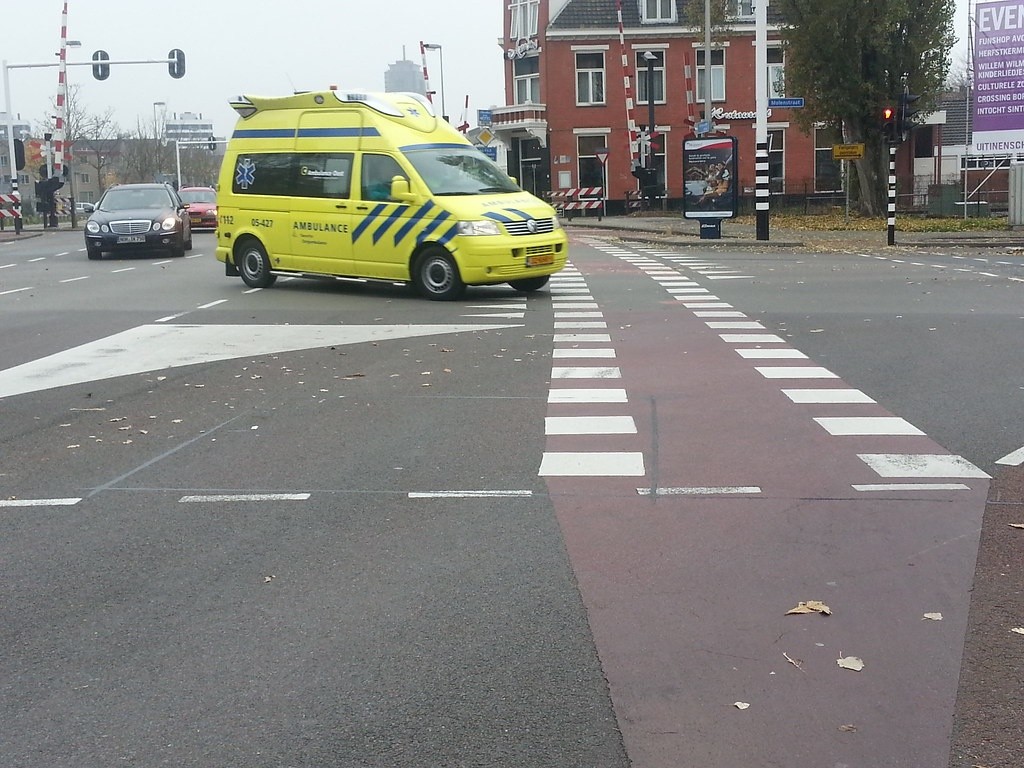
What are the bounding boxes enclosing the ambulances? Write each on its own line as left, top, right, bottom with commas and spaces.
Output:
216, 86, 568, 301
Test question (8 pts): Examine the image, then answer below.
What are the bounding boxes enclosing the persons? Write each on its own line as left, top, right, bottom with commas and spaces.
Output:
691, 162, 730, 206
366, 168, 399, 203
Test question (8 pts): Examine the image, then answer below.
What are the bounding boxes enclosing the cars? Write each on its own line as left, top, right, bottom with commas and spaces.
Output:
176, 185, 218, 231
84, 181, 192, 260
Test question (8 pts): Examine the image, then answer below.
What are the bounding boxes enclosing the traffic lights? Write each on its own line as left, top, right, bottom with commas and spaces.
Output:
883, 86, 922, 146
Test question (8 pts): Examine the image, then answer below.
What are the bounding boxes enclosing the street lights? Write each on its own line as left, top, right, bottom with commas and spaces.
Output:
154, 102, 166, 163
65, 41, 82, 230
423, 44, 445, 118
